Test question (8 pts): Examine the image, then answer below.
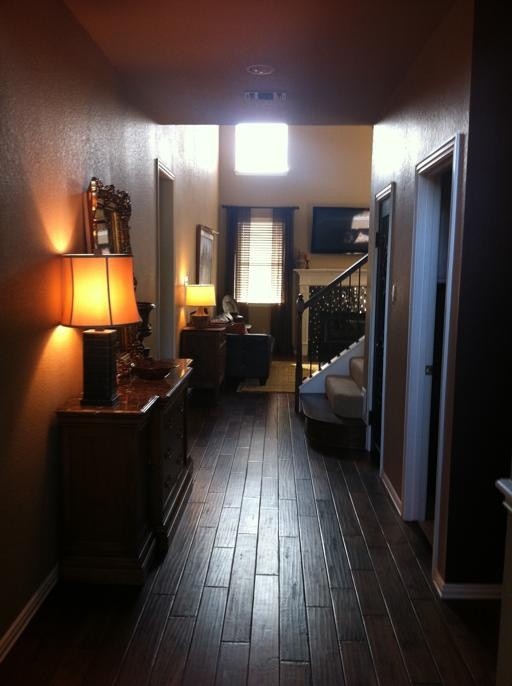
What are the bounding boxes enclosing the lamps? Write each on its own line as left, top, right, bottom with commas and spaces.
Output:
186, 284, 216, 329
62, 250, 141, 410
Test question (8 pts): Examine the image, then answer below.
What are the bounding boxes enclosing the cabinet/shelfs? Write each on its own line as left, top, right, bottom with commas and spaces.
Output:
181, 325, 227, 399
54, 357, 194, 602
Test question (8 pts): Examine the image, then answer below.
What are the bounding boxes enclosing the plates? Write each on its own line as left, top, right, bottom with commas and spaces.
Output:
222, 294, 238, 312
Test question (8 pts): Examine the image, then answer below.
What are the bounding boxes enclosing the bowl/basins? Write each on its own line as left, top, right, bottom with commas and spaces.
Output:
129, 359, 174, 380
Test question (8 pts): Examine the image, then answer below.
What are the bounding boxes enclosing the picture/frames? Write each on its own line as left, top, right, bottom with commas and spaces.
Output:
196, 224, 216, 285
